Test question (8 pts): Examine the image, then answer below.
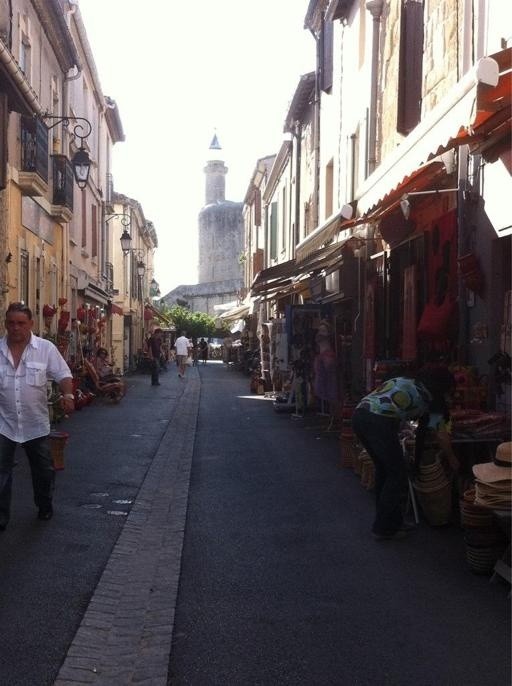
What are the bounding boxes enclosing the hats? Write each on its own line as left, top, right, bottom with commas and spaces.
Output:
471, 442, 512, 512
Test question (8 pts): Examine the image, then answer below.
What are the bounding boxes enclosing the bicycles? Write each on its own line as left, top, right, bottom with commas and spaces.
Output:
192, 347, 202, 367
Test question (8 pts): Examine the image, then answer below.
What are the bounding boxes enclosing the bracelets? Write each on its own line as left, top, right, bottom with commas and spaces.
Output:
63, 394, 75, 400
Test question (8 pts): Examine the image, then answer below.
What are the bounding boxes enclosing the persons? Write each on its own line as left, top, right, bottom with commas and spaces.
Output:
94, 348, 113, 377
352, 367, 455, 540
147, 329, 164, 385
192, 338, 208, 367
173, 331, 190, 379
223, 332, 232, 367
0, 302, 75, 532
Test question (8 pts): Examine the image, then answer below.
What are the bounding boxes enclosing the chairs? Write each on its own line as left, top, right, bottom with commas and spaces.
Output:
82, 357, 124, 409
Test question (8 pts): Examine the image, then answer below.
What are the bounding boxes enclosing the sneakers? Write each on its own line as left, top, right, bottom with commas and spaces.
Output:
372, 524, 416, 540
0, 508, 54, 530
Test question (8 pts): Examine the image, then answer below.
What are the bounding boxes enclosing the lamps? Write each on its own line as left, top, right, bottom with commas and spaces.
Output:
103, 208, 161, 297
41, 112, 92, 191
